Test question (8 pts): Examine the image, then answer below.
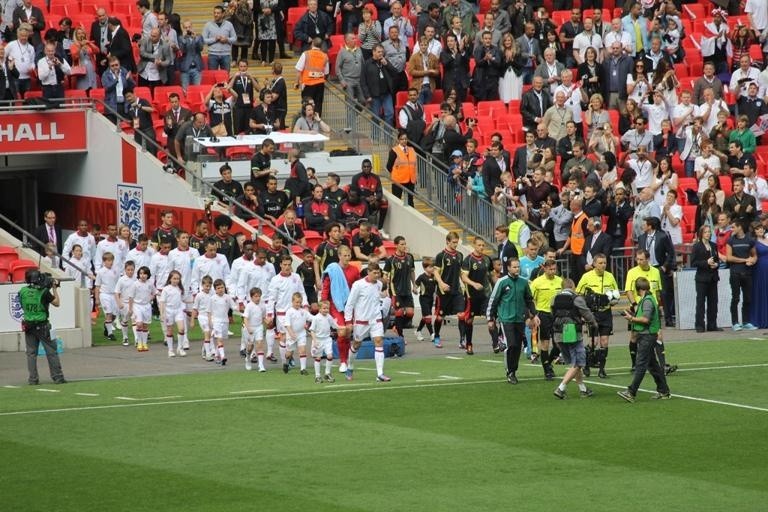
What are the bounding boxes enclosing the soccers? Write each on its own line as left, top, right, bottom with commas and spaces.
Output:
606, 289, 620, 305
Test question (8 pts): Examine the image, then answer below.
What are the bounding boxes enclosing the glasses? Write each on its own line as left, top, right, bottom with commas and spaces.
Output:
635, 122, 642, 125
636, 65, 644, 68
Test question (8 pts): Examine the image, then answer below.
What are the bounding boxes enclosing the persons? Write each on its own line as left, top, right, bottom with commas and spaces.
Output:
0, 0, 767, 402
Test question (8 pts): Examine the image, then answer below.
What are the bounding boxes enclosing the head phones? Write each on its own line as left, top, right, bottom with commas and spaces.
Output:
636, 277, 650, 291
260, 88, 268, 100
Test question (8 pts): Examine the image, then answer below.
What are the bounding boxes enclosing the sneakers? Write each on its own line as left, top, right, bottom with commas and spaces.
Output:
163, 337, 190, 358
492, 340, 507, 355
240, 348, 277, 373
458, 340, 473, 355
662, 364, 678, 375
528, 353, 608, 379
554, 388, 570, 399
283, 356, 391, 385
651, 393, 672, 400
578, 389, 595, 398
90, 318, 152, 352
201, 349, 228, 366
414, 329, 442, 348
616, 390, 636, 404
506, 371, 519, 385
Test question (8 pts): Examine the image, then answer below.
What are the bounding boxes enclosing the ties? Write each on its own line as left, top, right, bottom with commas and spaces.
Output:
414, 104, 418, 115
50, 227, 54, 243
174, 112, 178, 123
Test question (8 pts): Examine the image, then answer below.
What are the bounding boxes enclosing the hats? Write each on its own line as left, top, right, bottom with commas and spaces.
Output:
666, 15, 682, 31
751, 82, 759, 88
473, 158, 485, 167
452, 150, 462, 157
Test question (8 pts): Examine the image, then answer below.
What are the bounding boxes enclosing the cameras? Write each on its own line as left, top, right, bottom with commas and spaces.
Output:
188, 30, 192, 35
574, 191, 579, 195
537, 150, 543, 154
53, 59, 57, 63
598, 127, 604, 130
649, 92, 655, 95
466, 118, 478, 126
240, 72, 246, 76
217, 83, 224, 87
527, 175, 532, 178
